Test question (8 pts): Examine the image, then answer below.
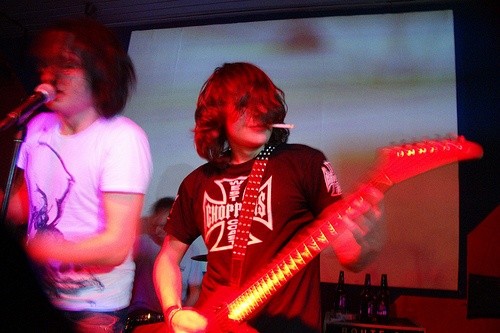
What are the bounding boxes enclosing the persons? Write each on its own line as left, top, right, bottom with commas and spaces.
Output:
0, 15, 155, 333
151, 62, 386, 333
131, 196, 204, 316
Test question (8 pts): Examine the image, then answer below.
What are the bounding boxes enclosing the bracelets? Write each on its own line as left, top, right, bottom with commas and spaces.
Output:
164, 305, 181, 331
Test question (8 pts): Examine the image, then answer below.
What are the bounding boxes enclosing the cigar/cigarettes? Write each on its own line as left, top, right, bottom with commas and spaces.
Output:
269, 122, 295, 130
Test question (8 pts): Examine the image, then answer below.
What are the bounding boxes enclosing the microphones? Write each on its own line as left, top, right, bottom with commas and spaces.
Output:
0, 84, 56, 135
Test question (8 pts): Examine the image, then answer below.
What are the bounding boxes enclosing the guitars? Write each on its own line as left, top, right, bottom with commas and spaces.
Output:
122, 133, 484, 333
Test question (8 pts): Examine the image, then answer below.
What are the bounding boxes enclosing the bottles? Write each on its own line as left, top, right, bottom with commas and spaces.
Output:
358, 274, 375, 323
332, 270, 346, 320
375, 274, 392, 325
